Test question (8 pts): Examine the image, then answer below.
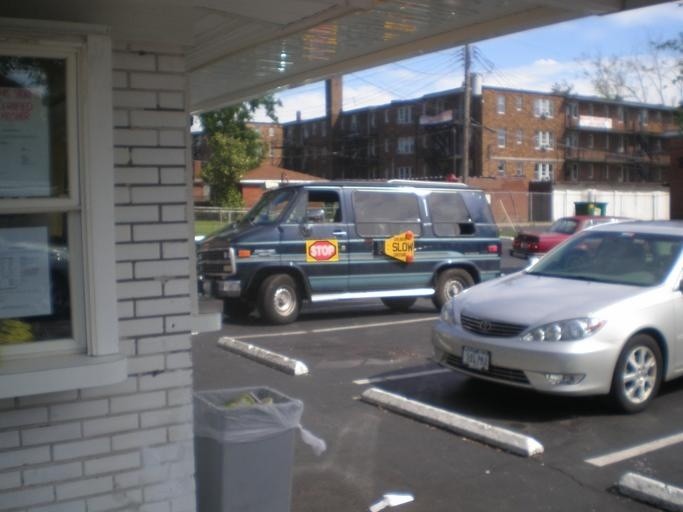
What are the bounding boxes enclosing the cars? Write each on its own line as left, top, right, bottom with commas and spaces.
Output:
508, 214, 619, 262
429, 217, 682, 414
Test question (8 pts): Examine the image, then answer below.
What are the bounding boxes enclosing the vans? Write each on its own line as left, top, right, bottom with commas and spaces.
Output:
197, 175, 504, 328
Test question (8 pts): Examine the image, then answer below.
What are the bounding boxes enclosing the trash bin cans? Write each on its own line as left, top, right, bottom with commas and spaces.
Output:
575, 201, 609, 217
192, 384, 305, 511
